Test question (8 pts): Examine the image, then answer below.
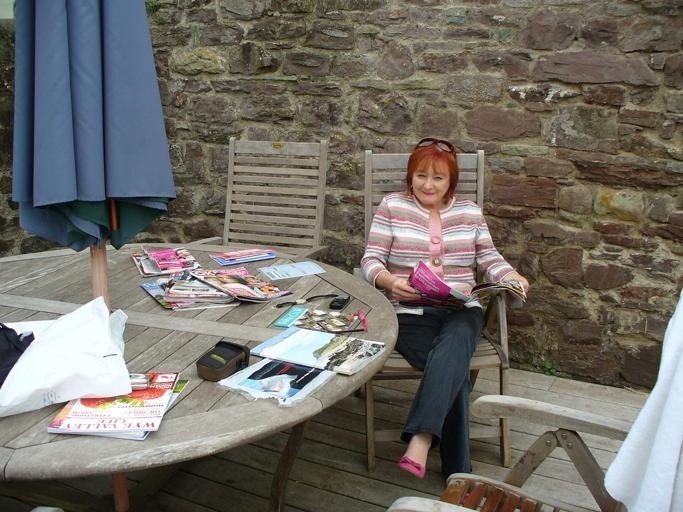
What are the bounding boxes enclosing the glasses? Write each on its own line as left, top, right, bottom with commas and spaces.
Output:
414, 137, 452, 153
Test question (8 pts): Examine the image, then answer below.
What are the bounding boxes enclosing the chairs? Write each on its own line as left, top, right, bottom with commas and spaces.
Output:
353, 151, 511, 473
386, 395, 632, 512
189, 137, 328, 259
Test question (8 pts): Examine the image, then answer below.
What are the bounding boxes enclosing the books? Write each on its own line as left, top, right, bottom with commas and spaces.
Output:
399, 261, 526, 313
266, 305, 361, 335
131, 244, 326, 312
48, 379, 190, 440
59, 371, 180, 431
249, 326, 387, 376
218, 359, 336, 407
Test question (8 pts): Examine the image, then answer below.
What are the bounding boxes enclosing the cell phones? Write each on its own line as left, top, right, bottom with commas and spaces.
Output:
329, 294, 350, 309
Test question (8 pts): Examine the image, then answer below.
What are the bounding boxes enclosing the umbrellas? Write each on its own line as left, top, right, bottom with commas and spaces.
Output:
11, 1, 177, 313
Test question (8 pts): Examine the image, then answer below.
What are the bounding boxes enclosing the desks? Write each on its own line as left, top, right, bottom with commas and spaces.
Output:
0, 242, 399, 512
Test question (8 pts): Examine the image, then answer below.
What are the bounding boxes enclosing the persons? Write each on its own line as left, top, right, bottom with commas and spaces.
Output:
360, 138, 529, 504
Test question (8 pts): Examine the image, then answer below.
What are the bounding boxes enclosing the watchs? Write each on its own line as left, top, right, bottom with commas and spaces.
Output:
276, 293, 338, 308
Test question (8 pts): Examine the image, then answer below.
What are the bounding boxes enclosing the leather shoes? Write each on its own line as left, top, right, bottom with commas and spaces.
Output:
399, 454, 425, 478
